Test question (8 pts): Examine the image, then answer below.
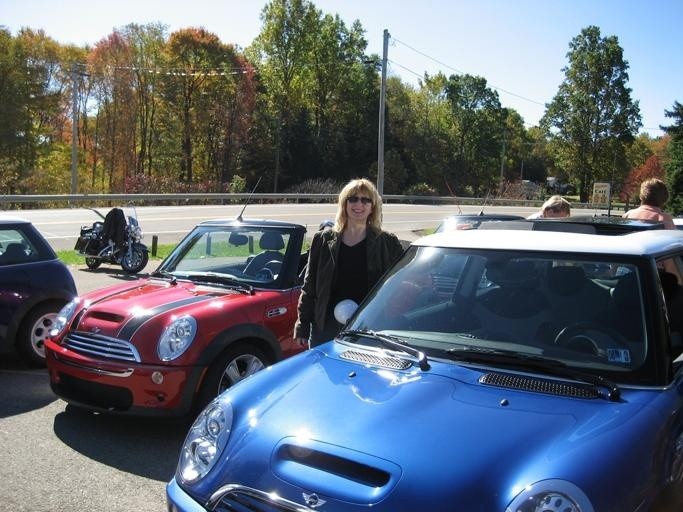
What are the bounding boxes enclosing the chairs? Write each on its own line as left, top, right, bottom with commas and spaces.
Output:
466, 264, 683, 365
0, 243, 30, 259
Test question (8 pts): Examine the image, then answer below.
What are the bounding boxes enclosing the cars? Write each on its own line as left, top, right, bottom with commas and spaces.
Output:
425, 211, 528, 302
0, 219, 80, 369
165, 214, 683, 512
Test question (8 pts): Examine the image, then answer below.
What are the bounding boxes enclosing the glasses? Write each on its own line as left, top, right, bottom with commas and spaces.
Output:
348, 195, 372, 204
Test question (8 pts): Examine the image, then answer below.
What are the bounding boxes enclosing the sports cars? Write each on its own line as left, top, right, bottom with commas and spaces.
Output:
42, 174, 337, 420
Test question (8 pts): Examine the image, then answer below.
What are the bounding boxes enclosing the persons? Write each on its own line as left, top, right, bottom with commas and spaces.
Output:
615, 177, 683, 284
293, 178, 410, 353
526, 194, 571, 219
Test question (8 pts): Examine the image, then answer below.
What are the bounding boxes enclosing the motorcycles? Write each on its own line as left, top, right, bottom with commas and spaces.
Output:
74, 205, 151, 274
